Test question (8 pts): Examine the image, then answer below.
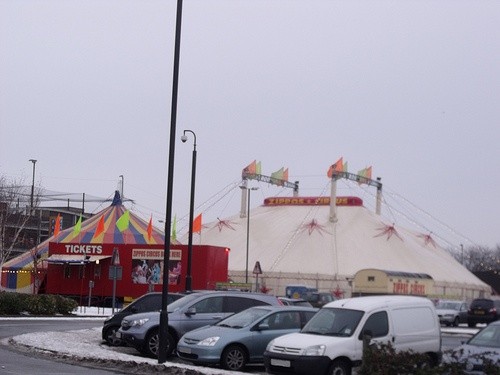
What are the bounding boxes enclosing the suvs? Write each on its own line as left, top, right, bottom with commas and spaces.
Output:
263, 295, 444, 375
118, 289, 292, 360
435, 299, 469, 328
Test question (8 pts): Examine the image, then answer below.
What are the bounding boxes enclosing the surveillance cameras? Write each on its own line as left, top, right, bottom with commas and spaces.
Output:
181, 136, 187, 142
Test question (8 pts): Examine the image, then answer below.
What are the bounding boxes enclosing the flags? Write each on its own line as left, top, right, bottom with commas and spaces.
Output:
171, 216, 176, 240
73, 214, 82, 236
53, 212, 61, 235
116, 209, 129, 233
146, 213, 153, 238
94, 214, 105, 238
241, 160, 289, 188
191, 214, 202, 234
326, 158, 372, 186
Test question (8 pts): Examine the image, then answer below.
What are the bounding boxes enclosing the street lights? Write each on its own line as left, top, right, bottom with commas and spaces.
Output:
27, 158, 37, 252
180, 129, 196, 294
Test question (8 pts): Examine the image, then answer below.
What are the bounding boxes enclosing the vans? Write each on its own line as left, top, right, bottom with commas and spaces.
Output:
466, 298, 500, 328
301, 291, 338, 308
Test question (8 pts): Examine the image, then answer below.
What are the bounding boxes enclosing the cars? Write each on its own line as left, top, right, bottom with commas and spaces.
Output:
443, 321, 500, 375
277, 295, 315, 308
176, 305, 337, 372
101, 292, 190, 347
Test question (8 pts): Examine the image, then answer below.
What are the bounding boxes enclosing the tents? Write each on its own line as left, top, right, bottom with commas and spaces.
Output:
0, 190, 227, 313
180, 193, 496, 314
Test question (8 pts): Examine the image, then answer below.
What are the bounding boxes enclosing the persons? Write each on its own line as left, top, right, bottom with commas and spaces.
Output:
133, 256, 181, 286
